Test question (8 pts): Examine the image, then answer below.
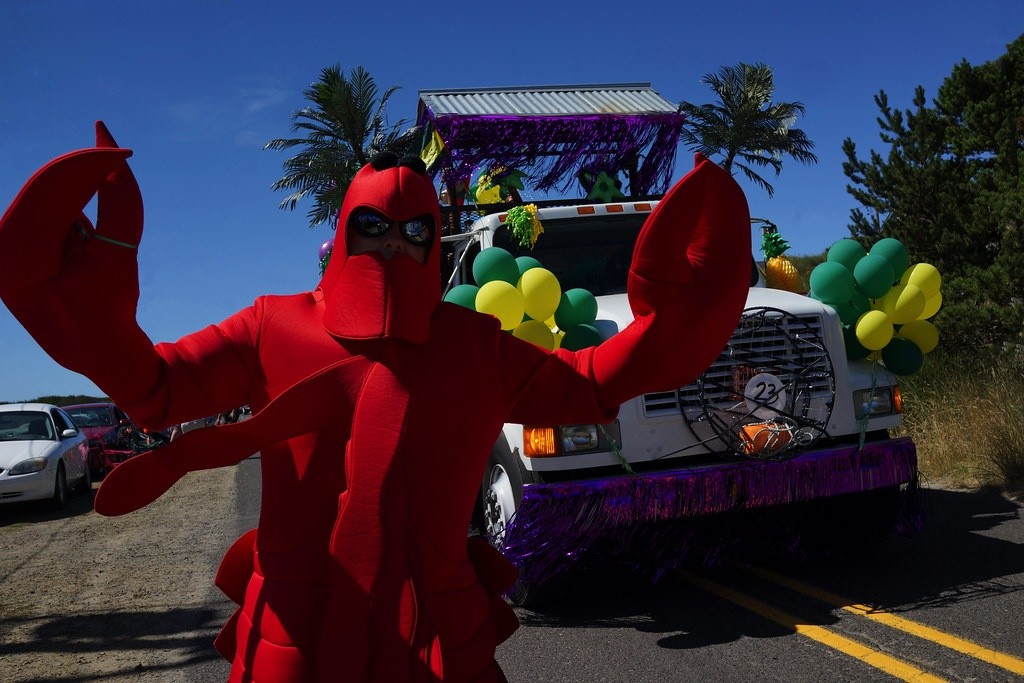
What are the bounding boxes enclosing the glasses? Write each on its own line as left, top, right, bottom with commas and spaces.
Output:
349, 211, 435, 247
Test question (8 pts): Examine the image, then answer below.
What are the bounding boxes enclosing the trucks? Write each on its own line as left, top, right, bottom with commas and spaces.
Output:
318, 81, 919, 588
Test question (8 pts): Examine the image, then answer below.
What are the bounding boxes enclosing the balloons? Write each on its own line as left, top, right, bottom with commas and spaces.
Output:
443, 246, 601, 352
807, 239, 943, 377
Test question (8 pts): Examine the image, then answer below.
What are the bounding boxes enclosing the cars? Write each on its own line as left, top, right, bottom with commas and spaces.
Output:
57, 402, 142, 480
241, 405, 252, 415
0, 401, 93, 507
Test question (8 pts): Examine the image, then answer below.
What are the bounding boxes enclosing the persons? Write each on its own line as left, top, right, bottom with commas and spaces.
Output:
0, 118, 752, 683
